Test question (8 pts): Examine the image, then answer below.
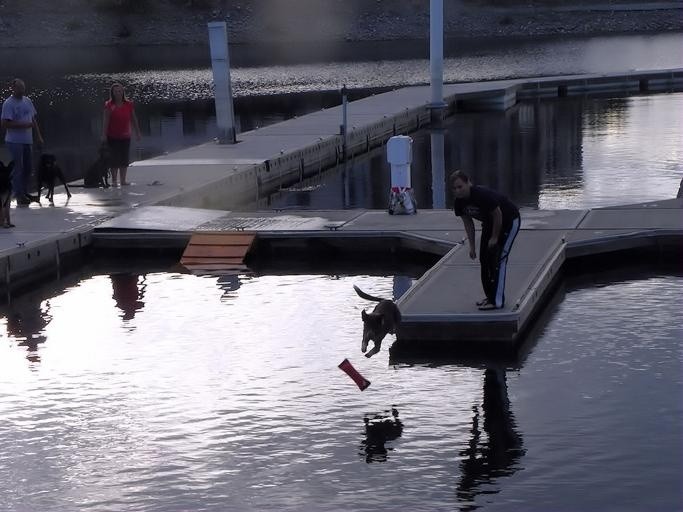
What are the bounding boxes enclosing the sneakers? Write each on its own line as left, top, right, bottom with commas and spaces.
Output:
476, 298, 496, 310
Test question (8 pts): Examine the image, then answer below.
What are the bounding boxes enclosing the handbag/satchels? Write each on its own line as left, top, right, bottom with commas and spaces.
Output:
389, 186, 417, 214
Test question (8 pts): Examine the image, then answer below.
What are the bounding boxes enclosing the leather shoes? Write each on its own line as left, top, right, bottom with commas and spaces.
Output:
17, 194, 38, 204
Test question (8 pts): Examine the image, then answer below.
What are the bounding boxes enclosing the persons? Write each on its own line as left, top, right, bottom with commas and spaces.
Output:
103, 83, 141, 187
448, 169, 521, 311
1, 79, 45, 204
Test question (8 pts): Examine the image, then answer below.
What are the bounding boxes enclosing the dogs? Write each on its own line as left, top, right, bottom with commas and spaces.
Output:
36, 153, 75, 202
0, 159, 16, 228
352, 284, 401, 358
67, 146, 112, 189
